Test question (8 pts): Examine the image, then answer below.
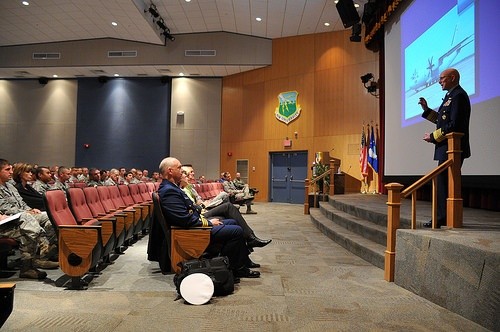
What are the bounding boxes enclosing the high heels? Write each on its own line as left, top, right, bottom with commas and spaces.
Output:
247, 232, 271, 251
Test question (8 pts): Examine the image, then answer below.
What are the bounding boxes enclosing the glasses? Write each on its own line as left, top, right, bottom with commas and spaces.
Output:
440, 76, 451, 80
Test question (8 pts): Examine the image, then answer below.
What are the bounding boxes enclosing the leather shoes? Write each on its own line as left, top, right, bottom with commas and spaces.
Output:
238, 268, 260, 278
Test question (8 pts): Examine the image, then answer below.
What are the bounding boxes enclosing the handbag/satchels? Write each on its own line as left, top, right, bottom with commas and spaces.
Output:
173, 253, 234, 300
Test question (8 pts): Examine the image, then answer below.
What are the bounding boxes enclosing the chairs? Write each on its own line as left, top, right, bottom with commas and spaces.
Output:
0, 180, 259, 291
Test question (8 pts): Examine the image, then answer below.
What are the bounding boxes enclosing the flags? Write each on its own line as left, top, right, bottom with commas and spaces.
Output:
360, 125, 380, 184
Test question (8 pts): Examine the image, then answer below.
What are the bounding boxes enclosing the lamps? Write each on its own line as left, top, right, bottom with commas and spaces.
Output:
359, 72, 378, 92
143, 7, 175, 41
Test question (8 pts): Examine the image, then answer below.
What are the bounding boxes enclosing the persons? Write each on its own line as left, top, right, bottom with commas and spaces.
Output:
0, 158, 162, 284
177, 164, 272, 267
154, 158, 260, 278
417, 69, 472, 228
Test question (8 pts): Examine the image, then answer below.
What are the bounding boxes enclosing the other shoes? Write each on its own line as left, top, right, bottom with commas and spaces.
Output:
423, 220, 446, 227
246, 256, 260, 268
20, 269, 47, 279
33, 259, 60, 269
39, 244, 56, 260
246, 210, 257, 214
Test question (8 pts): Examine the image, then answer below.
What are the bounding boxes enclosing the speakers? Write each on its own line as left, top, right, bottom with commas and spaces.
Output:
336, 0, 361, 29
